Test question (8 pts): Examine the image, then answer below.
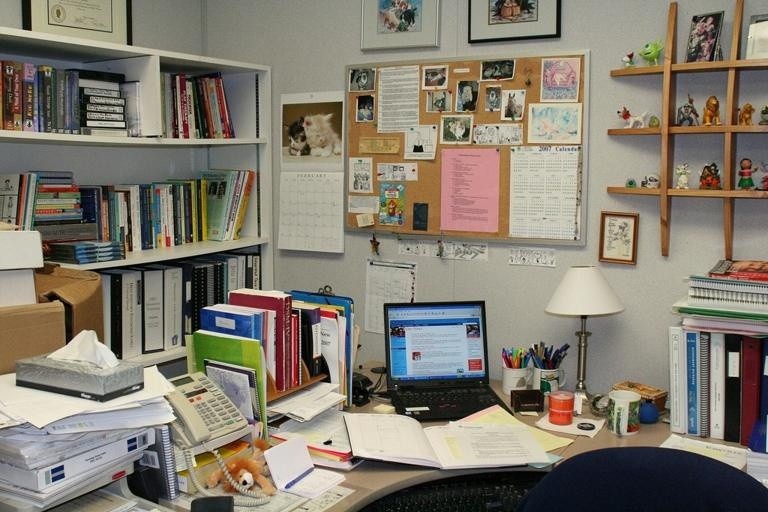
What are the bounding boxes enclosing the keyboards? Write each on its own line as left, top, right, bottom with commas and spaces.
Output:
376, 480, 533, 511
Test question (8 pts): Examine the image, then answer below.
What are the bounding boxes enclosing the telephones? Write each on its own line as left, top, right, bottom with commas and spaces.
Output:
164, 371, 270, 507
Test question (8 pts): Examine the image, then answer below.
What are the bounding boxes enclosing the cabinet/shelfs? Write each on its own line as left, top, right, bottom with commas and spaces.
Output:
605, 0, 768, 263
0, 26, 274, 372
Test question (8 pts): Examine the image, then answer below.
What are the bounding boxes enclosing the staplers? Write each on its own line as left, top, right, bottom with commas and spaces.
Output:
352, 372, 372, 407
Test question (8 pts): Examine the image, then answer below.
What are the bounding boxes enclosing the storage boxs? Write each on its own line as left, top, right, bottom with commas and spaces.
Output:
0, 262, 104, 375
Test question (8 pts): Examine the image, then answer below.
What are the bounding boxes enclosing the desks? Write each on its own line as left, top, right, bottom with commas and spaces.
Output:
48, 362, 760, 511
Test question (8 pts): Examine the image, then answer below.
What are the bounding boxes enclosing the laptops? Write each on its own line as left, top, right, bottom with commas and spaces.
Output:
383, 300, 515, 420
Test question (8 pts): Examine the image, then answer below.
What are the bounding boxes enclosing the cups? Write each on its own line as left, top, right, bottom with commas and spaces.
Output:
606, 390, 641, 436
502, 368, 533, 394
533, 369, 566, 395
550, 393, 573, 425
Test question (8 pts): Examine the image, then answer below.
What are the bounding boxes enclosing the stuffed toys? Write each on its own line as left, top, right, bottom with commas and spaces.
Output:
204, 439, 276, 496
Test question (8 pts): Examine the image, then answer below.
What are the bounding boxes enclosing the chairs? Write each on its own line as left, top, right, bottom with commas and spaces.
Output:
523, 445, 761, 510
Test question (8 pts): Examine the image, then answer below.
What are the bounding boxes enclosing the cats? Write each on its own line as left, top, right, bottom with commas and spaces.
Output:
283, 116, 311, 156
303, 111, 342, 158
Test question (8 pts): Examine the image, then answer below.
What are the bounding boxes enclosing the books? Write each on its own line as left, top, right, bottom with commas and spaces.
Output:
160, 70, 235, 139
0, 169, 255, 265
0, 59, 141, 139
99, 250, 261, 362
176, 441, 252, 494
193, 288, 347, 403
669, 259, 768, 452
270, 411, 366, 470
342, 413, 529, 470
1, 412, 176, 511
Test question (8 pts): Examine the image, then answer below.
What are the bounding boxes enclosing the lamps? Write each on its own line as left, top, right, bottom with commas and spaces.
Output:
543, 266, 626, 407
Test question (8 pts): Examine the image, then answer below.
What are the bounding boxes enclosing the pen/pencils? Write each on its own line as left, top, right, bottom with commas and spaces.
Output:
529, 341, 570, 369
502, 347, 531, 368
285, 466, 316, 489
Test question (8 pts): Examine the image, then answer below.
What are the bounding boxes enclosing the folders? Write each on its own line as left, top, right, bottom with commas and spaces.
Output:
742, 337, 761, 446
725, 334, 741, 442
760, 337, 768, 454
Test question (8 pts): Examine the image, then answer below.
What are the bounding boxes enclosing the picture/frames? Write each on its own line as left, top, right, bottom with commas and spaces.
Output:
359, 0, 439, 50
467, 0, 561, 44
598, 211, 639, 266
